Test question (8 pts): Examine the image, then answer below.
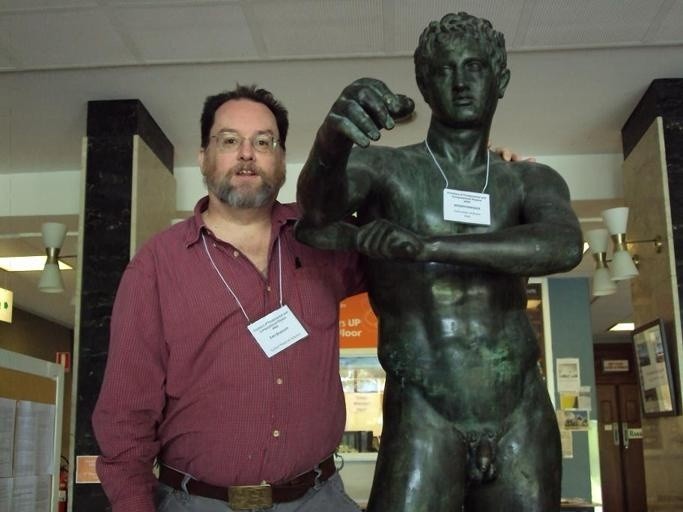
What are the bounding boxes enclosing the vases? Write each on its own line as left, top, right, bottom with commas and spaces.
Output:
631, 318, 679, 420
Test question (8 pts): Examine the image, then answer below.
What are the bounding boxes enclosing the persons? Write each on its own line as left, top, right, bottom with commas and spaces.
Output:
292, 11, 584, 512
90, 83, 536, 512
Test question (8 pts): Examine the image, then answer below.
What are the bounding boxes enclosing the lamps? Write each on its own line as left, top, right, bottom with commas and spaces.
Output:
36, 222, 76, 292
584, 205, 662, 297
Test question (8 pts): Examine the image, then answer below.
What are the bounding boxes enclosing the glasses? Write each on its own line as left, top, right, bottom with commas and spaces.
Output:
211, 131, 282, 152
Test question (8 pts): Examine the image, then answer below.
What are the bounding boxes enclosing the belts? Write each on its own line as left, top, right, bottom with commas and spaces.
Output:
159, 453, 337, 510
